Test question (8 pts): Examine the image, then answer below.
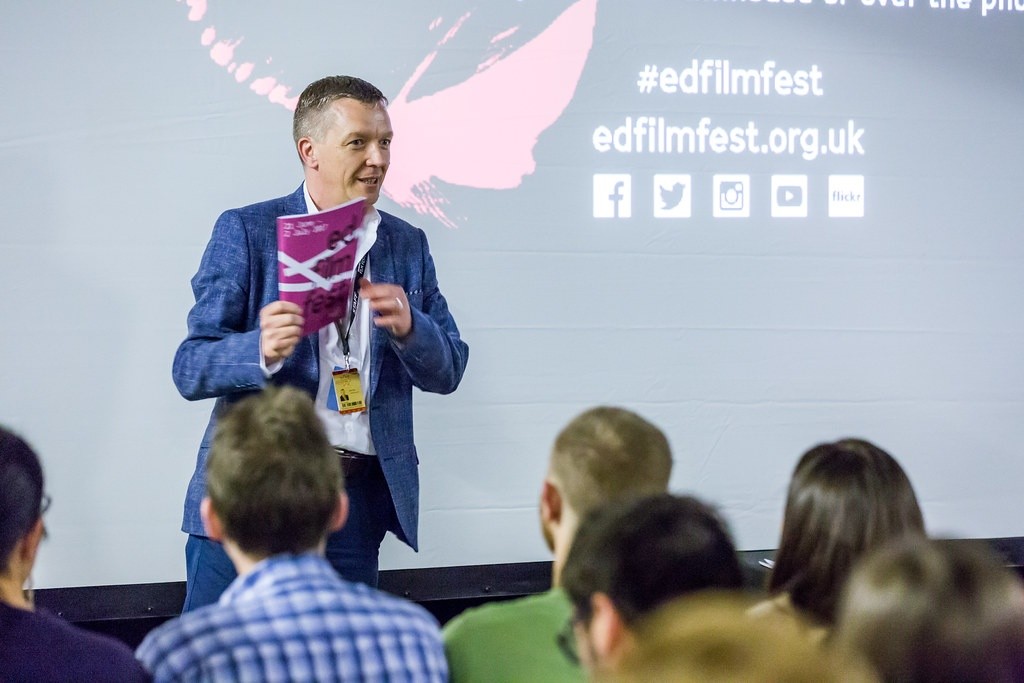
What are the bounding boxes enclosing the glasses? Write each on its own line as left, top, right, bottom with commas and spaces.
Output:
26, 494, 50, 538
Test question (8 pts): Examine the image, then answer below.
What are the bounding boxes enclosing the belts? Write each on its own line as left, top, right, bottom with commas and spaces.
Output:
336, 449, 379, 478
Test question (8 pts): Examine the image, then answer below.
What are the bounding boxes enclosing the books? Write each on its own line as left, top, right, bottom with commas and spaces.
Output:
274, 196, 368, 341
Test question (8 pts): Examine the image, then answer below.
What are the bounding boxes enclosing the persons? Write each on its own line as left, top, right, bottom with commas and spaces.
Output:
0, 427, 159, 683
169, 75, 470, 627
134, 388, 452, 682
440, 404, 1024, 683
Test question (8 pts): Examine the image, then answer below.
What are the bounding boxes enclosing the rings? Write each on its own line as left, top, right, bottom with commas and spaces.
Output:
396, 296, 404, 313
